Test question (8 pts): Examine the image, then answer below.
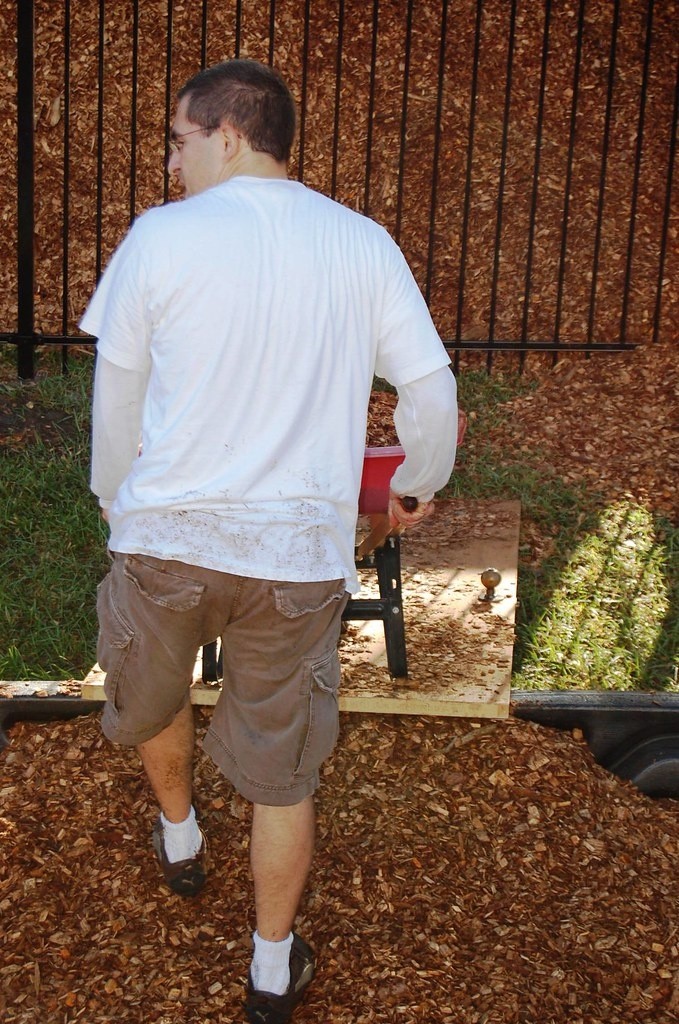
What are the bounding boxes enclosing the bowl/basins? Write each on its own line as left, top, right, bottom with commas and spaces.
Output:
358, 445, 468, 514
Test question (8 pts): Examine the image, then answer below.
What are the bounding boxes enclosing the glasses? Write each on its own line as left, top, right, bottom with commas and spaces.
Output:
169, 125, 242, 153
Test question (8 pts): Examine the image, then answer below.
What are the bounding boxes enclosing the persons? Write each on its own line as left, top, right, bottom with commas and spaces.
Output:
78, 58, 458, 1024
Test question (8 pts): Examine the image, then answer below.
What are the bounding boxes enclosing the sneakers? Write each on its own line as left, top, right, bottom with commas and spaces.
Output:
247, 935, 316, 1024
152, 813, 209, 897
